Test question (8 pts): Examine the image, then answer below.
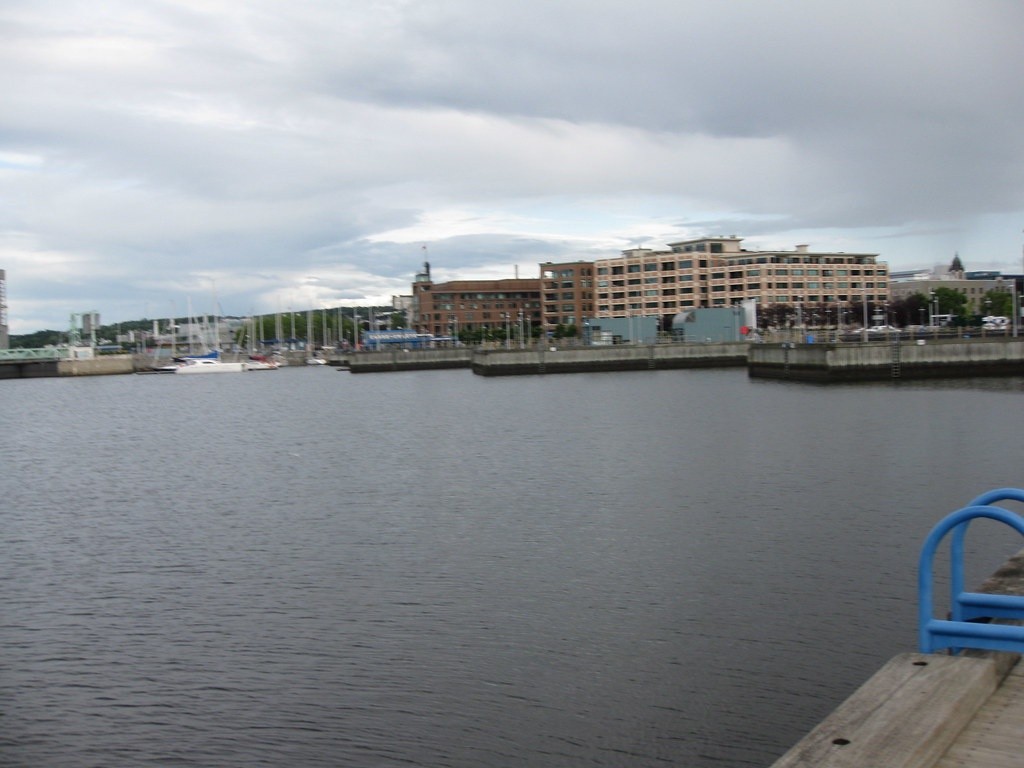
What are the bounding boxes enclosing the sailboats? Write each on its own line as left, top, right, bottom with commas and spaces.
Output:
141, 291, 362, 374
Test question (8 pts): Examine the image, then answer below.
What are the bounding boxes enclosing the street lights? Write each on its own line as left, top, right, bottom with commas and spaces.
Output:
584, 322, 590, 346
1008, 285, 1018, 338
824, 310, 832, 343
883, 303, 890, 341
